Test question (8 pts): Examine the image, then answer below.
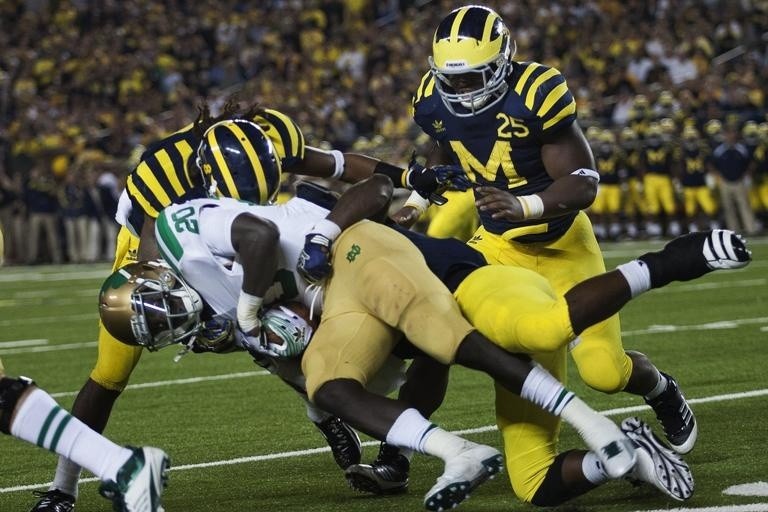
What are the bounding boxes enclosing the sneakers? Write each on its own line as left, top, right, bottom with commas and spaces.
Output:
621, 416, 695, 501
644, 371, 697, 455
596, 421, 637, 480
314, 417, 361, 470
423, 446, 505, 512
31, 489, 76, 511
666, 229, 752, 282
345, 442, 410, 494
99, 445, 172, 512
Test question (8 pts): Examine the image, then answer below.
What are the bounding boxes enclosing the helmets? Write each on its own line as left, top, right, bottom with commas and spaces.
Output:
428, 5, 517, 117
196, 118, 281, 206
231, 106, 305, 204
98, 262, 203, 352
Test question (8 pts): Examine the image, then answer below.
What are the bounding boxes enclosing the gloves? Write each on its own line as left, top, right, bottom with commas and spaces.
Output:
262, 305, 314, 359
296, 233, 333, 286
194, 319, 237, 353
239, 329, 279, 368
405, 167, 472, 206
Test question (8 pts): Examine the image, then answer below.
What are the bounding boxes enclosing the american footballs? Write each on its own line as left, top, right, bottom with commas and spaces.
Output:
261, 302, 316, 345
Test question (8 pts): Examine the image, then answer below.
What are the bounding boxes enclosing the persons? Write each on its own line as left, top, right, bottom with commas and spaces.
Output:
392, 8, 702, 509
0, 89, 751, 512
0, 0, 767, 271
